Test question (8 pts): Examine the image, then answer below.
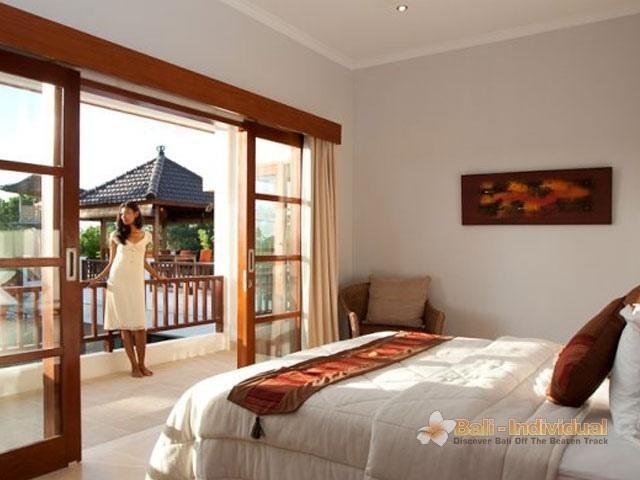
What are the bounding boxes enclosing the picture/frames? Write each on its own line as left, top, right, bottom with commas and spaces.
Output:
458, 164, 613, 227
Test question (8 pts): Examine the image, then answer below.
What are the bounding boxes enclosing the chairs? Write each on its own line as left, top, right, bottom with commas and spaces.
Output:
337, 282, 449, 339
152, 248, 212, 291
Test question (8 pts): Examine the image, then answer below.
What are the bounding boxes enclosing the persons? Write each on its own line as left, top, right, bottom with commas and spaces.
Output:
81, 199, 169, 379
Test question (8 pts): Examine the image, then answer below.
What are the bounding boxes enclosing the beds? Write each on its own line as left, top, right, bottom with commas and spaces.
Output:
175, 327, 640, 480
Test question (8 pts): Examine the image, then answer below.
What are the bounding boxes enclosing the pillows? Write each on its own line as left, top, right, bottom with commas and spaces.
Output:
548, 295, 640, 444
362, 271, 431, 329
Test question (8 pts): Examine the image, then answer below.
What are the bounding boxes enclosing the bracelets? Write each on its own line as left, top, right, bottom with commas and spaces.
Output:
95, 275, 102, 280
